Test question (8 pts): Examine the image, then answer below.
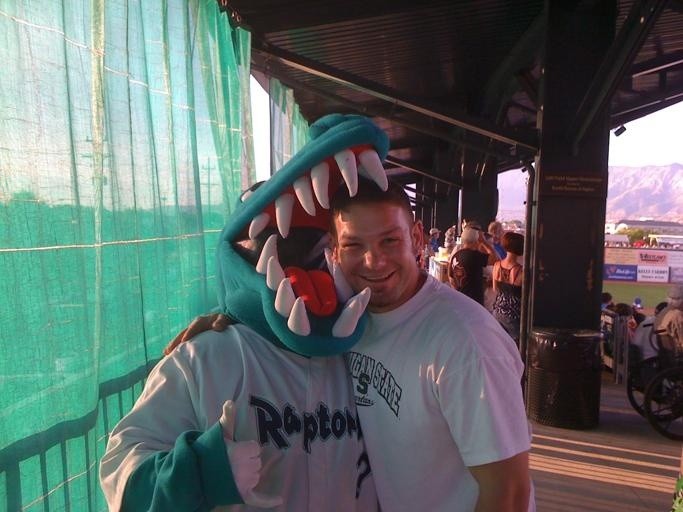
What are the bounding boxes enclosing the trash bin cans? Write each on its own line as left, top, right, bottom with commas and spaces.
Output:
527, 327, 605, 430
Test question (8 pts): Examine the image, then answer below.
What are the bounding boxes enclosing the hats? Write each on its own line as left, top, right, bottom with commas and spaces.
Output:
429, 228, 441, 235
668, 285, 683, 298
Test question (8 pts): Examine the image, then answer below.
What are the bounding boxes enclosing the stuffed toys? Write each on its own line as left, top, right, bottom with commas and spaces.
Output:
98, 113, 398, 512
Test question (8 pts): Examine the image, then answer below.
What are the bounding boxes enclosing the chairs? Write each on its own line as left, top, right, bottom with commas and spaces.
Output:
599, 308, 683, 441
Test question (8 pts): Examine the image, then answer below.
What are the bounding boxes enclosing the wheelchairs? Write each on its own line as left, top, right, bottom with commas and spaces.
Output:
626, 322, 683, 443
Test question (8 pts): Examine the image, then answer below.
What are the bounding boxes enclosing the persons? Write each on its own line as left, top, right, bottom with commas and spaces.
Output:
423, 216, 527, 350
602, 282, 683, 390
328, 181, 536, 512
